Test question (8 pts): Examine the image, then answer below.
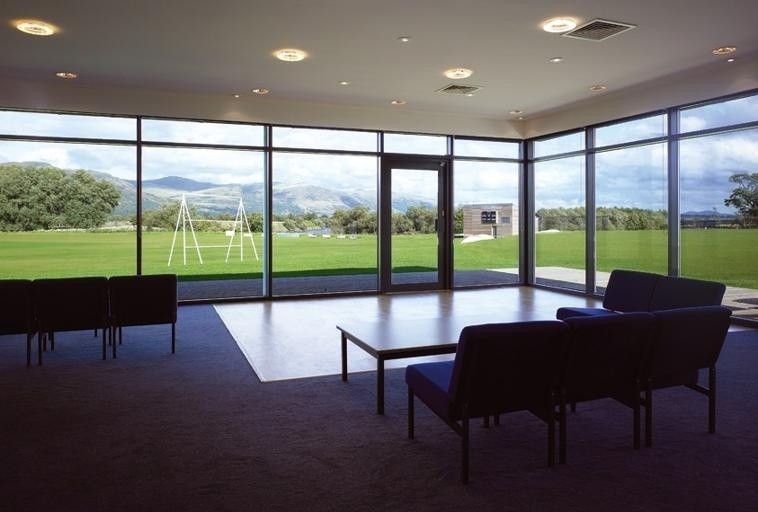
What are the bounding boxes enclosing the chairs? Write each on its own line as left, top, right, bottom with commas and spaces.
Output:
1, 272, 180, 368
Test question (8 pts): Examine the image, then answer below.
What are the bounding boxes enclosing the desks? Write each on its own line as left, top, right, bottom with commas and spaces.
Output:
333, 307, 565, 421
